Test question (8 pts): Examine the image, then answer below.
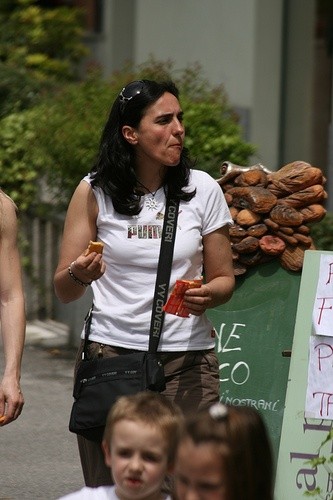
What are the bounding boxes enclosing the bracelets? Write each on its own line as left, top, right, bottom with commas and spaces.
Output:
55, 80, 235, 487
68, 266, 92, 289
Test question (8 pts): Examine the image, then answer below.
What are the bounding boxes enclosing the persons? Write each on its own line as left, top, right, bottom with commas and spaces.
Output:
62, 395, 182, 499
174, 404, 271, 500
1, 187, 24, 426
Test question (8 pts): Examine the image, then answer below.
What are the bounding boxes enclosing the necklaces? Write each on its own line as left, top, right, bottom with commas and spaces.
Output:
146, 197, 159, 211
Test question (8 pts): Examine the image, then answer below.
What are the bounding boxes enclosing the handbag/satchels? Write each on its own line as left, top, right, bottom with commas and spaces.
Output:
69, 352, 167, 442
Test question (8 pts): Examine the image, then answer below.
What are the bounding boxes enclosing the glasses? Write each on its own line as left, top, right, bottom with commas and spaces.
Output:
120, 80, 145, 117
